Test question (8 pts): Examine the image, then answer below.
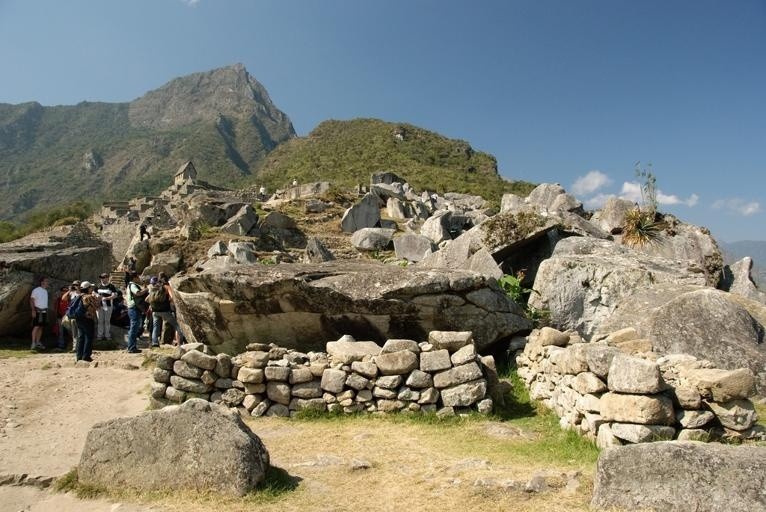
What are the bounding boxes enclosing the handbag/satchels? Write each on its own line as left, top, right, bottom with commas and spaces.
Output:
133, 293, 149, 313
65, 295, 83, 319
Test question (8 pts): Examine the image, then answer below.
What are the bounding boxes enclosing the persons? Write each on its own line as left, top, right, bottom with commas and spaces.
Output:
139, 222, 151, 241
252, 179, 298, 197
29, 254, 187, 362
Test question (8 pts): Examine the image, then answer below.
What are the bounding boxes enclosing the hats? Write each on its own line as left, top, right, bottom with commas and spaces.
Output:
99, 273, 108, 278
126, 270, 137, 278
71, 280, 95, 290
150, 272, 169, 284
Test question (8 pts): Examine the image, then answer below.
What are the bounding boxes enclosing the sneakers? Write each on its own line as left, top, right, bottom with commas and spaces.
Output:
128, 348, 141, 353
81, 355, 93, 362
96, 335, 113, 342
30, 342, 46, 350
